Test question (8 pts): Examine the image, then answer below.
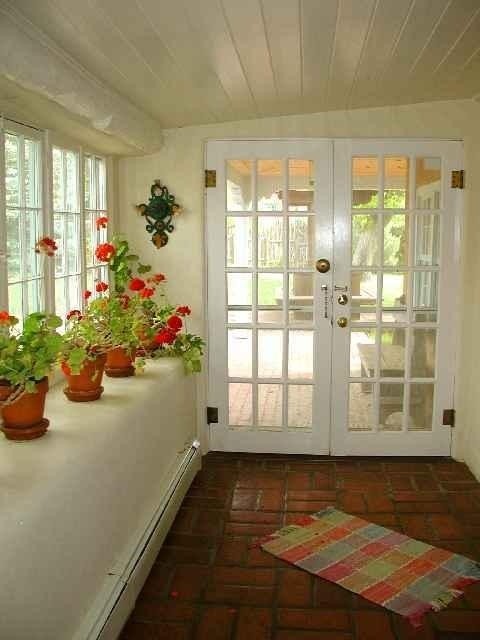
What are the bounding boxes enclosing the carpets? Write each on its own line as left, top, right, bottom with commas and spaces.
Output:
247, 506, 480, 630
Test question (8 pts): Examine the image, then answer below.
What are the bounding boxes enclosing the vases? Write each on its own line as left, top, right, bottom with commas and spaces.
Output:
61, 353, 107, 402
1, 376, 49, 441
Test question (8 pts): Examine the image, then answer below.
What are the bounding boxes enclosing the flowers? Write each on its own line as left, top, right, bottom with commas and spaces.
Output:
0, 216, 205, 393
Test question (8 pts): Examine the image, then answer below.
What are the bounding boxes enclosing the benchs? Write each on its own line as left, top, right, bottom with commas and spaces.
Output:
356, 342, 426, 429
